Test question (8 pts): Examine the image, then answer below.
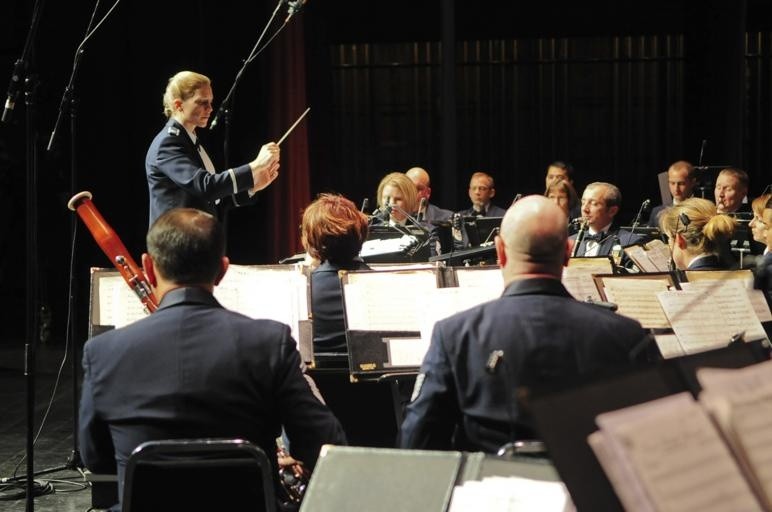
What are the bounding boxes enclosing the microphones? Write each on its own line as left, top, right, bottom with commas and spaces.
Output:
670, 212, 690, 269
570, 217, 584, 229
374, 202, 430, 235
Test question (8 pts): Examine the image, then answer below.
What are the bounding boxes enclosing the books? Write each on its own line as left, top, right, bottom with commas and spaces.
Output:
302, 443, 579, 512
576, 355, 772, 511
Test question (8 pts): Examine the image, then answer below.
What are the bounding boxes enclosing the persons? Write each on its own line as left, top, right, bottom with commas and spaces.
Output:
400, 193, 693, 511
143, 70, 282, 256
299, 160, 771, 368
75, 206, 350, 512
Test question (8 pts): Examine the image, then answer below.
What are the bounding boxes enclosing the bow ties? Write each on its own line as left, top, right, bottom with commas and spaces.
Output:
584, 232, 603, 243
194, 137, 202, 150
471, 209, 486, 217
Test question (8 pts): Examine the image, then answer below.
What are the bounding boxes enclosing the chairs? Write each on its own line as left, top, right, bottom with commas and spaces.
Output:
122, 439, 278, 511
